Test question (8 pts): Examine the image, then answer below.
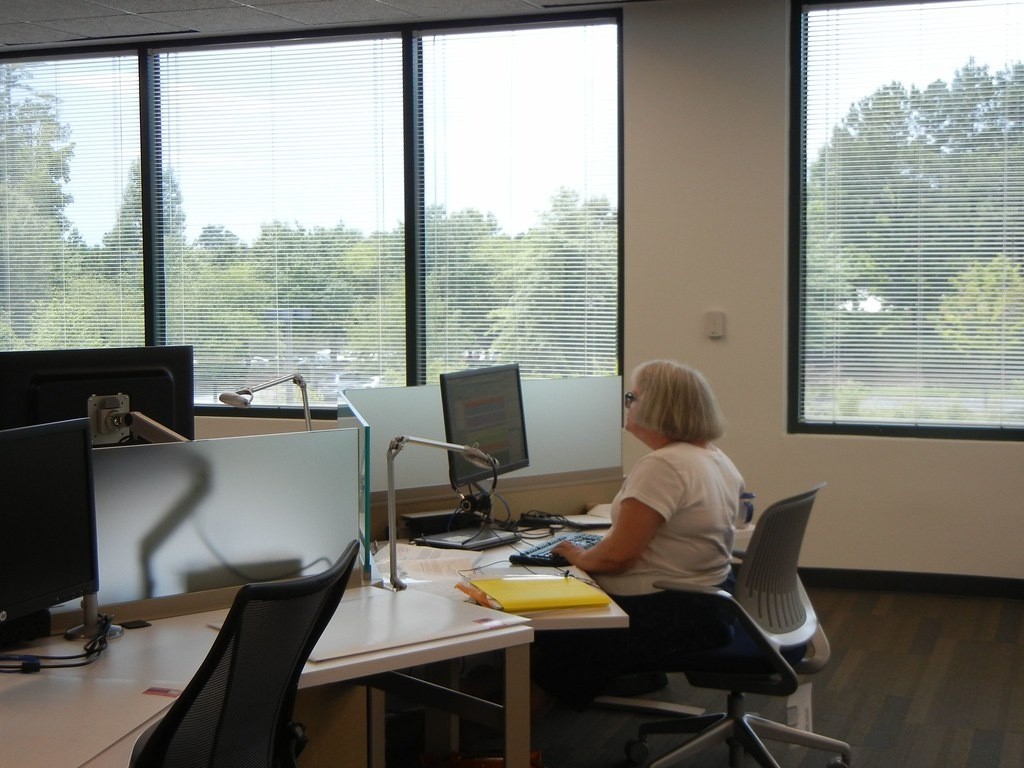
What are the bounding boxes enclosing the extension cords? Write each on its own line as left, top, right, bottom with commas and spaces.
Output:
519, 512, 564, 528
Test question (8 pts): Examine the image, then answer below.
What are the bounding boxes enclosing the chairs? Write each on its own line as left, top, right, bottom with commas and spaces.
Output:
625, 478, 850, 768
135, 538, 361, 768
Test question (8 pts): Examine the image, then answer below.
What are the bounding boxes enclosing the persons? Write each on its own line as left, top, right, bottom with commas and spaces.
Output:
534, 362, 745, 673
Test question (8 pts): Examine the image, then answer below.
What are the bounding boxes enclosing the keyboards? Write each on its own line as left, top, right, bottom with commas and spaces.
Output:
509, 534, 606, 567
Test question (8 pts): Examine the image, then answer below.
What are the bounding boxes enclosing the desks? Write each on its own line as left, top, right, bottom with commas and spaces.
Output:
0, 586, 534, 768
370, 515, 754, 755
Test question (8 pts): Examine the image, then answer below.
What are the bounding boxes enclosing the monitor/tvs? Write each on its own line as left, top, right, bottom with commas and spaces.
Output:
413, 364, 530, 550
0, 345, 195, 649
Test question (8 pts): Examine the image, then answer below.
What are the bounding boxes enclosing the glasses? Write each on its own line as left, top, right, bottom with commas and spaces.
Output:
625, 392, 635, 408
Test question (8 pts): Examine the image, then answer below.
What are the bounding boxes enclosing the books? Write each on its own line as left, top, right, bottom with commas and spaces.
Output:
469, 575, 611, 608
563, 504, 614, 528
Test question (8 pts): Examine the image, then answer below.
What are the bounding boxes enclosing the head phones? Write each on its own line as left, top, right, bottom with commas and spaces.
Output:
449, 450, 497, 512
